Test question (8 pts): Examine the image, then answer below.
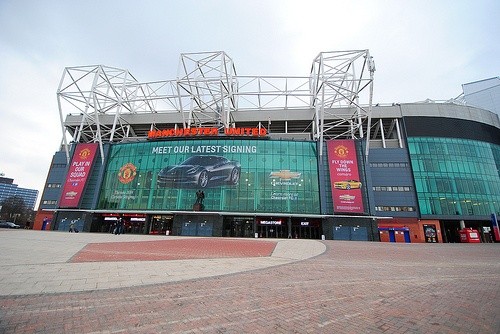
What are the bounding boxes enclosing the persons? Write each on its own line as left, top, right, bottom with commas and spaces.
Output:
234, 222, 255, 237
195, 189, 204, 203
68, 219, 76, 233
102, 218, 170, 235
24, 218, 33, 230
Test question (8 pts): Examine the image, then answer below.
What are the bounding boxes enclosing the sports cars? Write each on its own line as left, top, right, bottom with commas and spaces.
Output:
157, 155, 242, 189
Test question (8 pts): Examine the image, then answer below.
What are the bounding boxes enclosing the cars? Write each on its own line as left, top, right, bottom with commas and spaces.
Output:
0, 220, 10, 228
6, 222, 21, 229
